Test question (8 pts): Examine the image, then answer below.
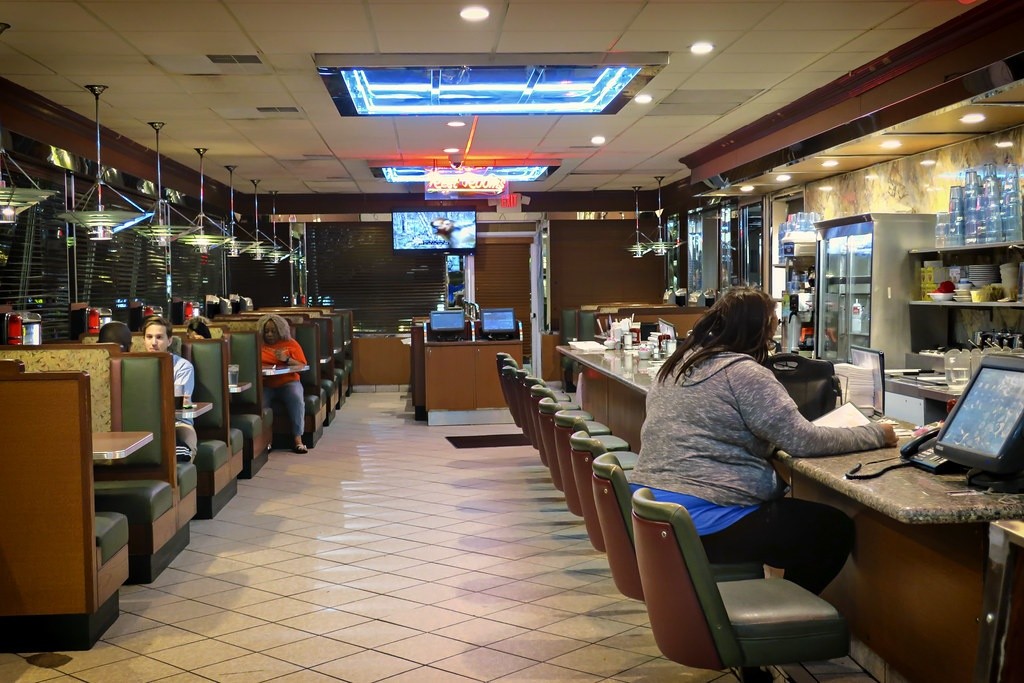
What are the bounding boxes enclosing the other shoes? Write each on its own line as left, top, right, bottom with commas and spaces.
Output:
741, 664, 792, 683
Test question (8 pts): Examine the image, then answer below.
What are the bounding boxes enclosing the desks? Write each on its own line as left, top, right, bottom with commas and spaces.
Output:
333, 347, 342, 354
174, 402, 213, 418
262, 368, 290, 375
320, 355, 332, 363
344, 340, 351, 345
230, 382, 252, 393
300, 365, 310, 371
93, 431, 153, 459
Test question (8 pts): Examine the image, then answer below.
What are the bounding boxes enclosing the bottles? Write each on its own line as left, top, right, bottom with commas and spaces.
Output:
184, 302, 199, 320
301, 296, 305, 304
144, 307, 162, 316
654, 334, 676, 360
788, 265, 816, 293
89, 310, 112, 333
616, 327, 640, 349
8, 315, 40, 345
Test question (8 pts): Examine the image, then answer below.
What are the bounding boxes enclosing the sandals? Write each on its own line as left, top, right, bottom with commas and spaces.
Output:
292, 444, 308, 453
268, 443, 272, 452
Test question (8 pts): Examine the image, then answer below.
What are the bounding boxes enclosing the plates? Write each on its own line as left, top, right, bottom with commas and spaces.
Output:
969, 265, 999, 287
953, 285, 972, 302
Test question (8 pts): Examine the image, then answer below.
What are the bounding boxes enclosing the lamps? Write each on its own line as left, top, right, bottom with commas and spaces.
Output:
245, 179, 283, 260
625, 184, 653, 258
0, 22, 57, 225
59, 84, 153, 241
263, 191, 293, 263
131, 120, 203, 246
221, 166, 264, 258
174, 145, 237, 254
645, 176, 682, 256
285, 192, 305, 265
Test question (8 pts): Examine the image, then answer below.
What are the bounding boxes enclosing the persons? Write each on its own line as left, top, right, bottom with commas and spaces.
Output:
95, 321, 134, 352
252, 314, 308, 455
143, 316, 197, 464
183, 315, 212, 324
626, 281, 900, 596
186, 321, 212, 340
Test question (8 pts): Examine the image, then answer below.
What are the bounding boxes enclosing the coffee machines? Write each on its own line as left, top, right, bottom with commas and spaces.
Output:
852, 299, 862, 332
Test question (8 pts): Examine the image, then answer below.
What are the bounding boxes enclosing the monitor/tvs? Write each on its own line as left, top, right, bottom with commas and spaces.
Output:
481, 308, 516, 333
391, 209, 478, 255
431, 310, 465, 333
934, 353, 1024, 495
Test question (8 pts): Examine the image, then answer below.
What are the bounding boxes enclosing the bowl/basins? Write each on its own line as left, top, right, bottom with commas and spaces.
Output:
604, 341, 614, 350
638, 351, 652, 359
927, 293, 955, 301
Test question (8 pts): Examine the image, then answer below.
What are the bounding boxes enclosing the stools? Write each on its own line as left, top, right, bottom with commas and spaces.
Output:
497, 348, 852, 683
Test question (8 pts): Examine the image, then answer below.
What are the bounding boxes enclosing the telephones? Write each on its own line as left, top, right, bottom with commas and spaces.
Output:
900, 427, 960, 475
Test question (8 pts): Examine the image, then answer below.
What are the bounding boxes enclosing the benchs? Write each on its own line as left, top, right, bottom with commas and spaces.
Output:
0, 307, 355, 649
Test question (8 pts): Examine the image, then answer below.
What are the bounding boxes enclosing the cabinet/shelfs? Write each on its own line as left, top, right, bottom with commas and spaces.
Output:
909, 241, 1024, 306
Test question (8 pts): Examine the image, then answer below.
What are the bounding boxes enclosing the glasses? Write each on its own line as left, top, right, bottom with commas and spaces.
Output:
767, 327, 778, 350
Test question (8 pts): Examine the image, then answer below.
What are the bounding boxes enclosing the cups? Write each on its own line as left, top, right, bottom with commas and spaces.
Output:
935, 164, 1024, 245
924, 261, 944, 283
946, 348, 1024, 391
970, 284, 1002, 302
1000, 263, 1018, 302
779, 212, 820, 233
228, 365, 239, 387
174, 385, 184, 411
779, 233, 786, 264
